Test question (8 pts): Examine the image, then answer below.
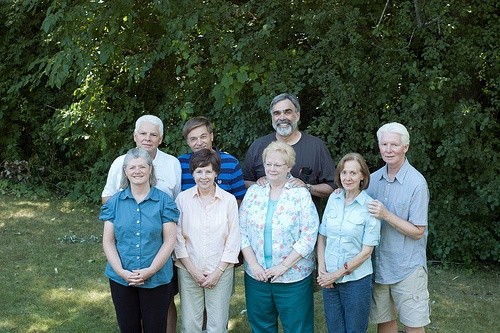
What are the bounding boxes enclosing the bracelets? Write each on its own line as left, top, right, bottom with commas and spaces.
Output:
216, 266, 225, 273
344, 262, 350, 275
306, 183, 311, 193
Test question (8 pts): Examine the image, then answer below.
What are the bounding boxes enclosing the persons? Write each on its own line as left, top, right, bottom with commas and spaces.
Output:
98, 92, 432, 333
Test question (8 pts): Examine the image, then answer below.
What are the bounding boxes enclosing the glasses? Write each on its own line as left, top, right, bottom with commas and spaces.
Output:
264, 163, 287, 168
